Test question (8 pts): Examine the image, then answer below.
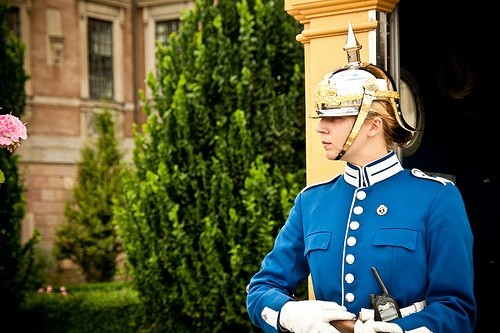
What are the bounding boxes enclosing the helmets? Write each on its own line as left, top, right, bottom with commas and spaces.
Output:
308, 60, 417, 143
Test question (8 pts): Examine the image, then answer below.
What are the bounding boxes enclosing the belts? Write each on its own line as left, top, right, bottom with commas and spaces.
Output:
399, 300, 428, 318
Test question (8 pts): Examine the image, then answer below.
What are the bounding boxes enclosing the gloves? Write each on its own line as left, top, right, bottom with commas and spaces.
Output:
354, 319, 403, 333
278, 300, 357, 333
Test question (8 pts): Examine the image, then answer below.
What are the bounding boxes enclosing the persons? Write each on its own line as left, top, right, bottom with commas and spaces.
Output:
245, 17, 477, 333
